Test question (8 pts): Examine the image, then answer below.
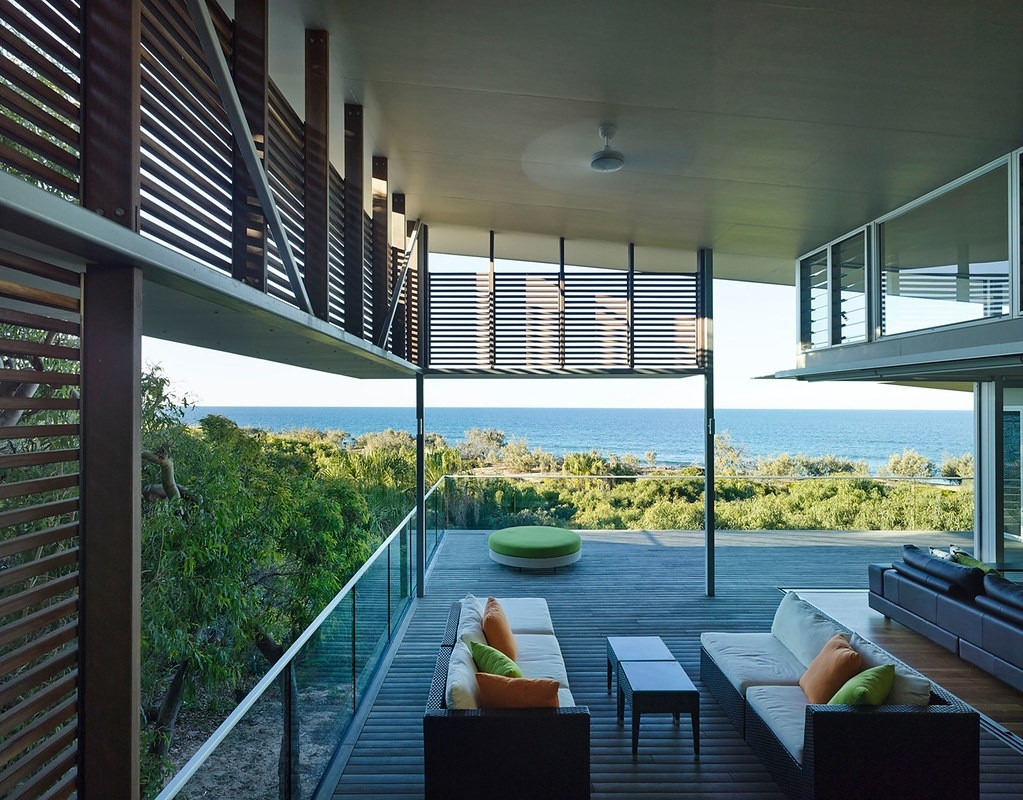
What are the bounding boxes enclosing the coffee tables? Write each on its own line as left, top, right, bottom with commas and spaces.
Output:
607, 636, 677, 724
618, 661, 700, 761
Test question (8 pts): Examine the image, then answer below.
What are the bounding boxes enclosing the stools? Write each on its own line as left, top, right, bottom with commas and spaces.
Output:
488, 526, 582, 573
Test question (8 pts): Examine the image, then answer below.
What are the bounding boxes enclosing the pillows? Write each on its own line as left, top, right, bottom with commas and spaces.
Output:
827, 664, 895, 709
798, 633, 863, 705
446, 638, 481, 708
480, 596, 517, 663
455, 592, 488, 651
956, 553, 1003, 577
470, 641, 524, 678
928, 546, 956, 564
770, 591, 851, 669
949, 544, 974, 563
474, 672, 560, 708
848, 631, 931, 707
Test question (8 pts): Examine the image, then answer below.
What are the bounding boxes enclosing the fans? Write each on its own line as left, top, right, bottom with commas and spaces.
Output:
521, 114, 697, 193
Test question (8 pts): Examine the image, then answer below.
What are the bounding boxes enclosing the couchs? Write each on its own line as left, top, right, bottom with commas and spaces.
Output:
868, 545, 1023, 694
422, 598, 590, 800
698, 599, 981, 800
